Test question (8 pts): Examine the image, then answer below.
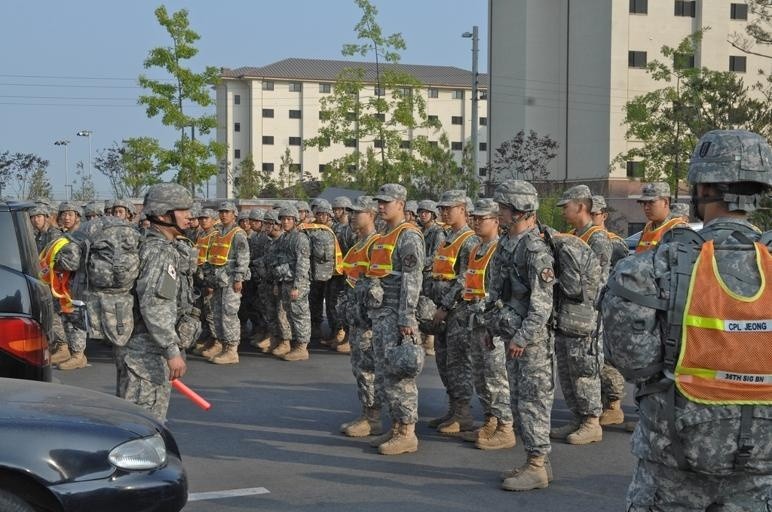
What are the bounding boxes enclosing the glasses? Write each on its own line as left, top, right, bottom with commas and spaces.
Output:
471, 216, 498, 222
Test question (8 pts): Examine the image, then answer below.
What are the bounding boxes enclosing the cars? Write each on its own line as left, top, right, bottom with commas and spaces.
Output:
0, 372, 189, 512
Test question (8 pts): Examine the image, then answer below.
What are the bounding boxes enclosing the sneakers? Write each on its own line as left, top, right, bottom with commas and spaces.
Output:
206, 344, 224, 363
56, 354, 72, 369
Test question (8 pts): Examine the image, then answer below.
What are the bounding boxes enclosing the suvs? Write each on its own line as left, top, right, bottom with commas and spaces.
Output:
0, 197, 55, 383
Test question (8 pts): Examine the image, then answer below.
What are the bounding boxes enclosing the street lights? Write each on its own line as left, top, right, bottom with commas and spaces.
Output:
460, 25, 479, 198
75, 129, 93, 198
52, 139, 69, 204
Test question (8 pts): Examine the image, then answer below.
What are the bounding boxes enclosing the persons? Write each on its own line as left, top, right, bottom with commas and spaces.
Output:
477, 180, 556, 490
624, 129, 771, 511
460, 198, 516, 450
425, 190, 478, 434
590, 194, 628, 427
339, 194, 384, 437
370, 183, 424, 455
28, 183, 687, 365
548, 184, 611, 445
115, 183, 188, 424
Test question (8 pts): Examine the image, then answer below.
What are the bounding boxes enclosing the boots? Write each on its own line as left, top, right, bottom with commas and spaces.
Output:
344, 406, 383, 439
281, 342, 309, 362
463, 417, 497, 443
377, 422, 419, 456
624, 419, 641, 432
511, 454, 554, 483
192, 338, 212, 355
473, 423, 517, 451
549, 415, 581, 440
320, 327, 352, 354
49, 343, 71, 366
366, 419, 395, 449
247, 331, 272, 348
436, 407, 475, 434
261, 339, 280, 354
419, 334, 436, 356
426, 404, 455, 429
339, 406, 365, 435
564, 417, 603, 446
58, 350, 88, 371
201, 339, 222, 357
599, 400, 626, 426
500, 455, 550, 492
271, 338, 291, 357
211, 343, 240, 365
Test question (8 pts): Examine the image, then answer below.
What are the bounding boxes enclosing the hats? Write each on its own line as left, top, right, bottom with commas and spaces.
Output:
555, 183, 595, 209
216, 200, 237, 213
196, 208, 219, 219
468, 197, 500, 216
434, 189, 468, 208
668, 202, 691, 219
635, 181, 672, 204
345, 194, 380, 214
371, 182, 408, 203
591, 195, 607, 213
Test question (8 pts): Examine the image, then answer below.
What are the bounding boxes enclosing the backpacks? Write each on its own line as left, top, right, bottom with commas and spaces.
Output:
609, 231, 631, 271
544, 231, 605, 339
46, 214, 145, 348
308, 222, 337, 285
657, 235, 772, 481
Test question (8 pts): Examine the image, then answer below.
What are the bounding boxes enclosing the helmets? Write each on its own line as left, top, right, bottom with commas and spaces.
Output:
414, 293, 447, 337
466, 196, 475, 213
310, 197, 323, 210
331, 196, 353, 211
271, 200, 286, 211
187, 201, 203, 219
29, 206, 51, 220
141, 181, 195, 218
687, 128, 772, 193
312, 200, 336, 217
56, 200, 83, 217
278, 203, 301, 225
416, 199, 441, 218
103, 199, 115, 211
293, 200, 310, 211
236, 210, 252, 224
492, 178, 540, 214
404, 200, 420, 216
263, 209, 283, 226
386, 334, 427, 380
111, 197, 137, 215
138, 208, 149, 223
83, 200, 105, 217
248, 206, 266, 223
28, 195, 51, 212
